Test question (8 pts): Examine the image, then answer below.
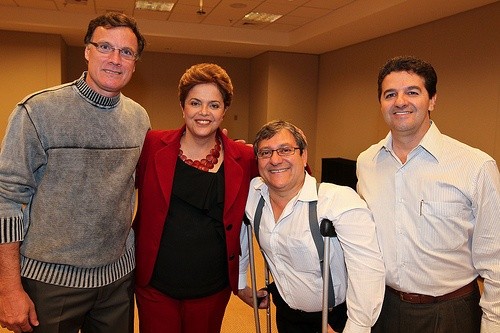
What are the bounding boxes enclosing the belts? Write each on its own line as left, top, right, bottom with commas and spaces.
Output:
385, 280, 477, 303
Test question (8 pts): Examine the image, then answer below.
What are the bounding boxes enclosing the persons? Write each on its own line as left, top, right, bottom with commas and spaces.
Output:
356, 56, 500, 333
237, 120, 386, 333
130, 64, 313, 333
0, 11, 254, 333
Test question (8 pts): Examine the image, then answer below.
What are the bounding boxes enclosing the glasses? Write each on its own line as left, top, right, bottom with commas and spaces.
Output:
89, 41, 139, 62
256, 146, 304, 158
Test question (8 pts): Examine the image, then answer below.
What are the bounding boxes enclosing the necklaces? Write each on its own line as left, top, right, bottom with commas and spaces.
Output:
178, 134, 221, 173
